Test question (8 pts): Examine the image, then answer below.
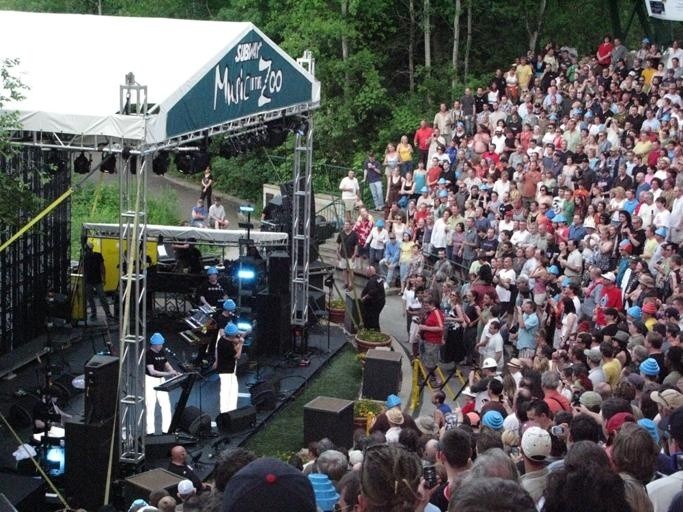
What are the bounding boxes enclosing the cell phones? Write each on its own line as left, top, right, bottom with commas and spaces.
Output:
552, 426, 564, 435
512, 445, 519, 456
423, 466, 437, 489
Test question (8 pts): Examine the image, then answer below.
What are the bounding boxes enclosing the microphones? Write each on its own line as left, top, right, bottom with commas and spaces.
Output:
235, 335, 245, 343
217, 283, 223, 289
165, 348, 176, 357
229, 312, 240, 319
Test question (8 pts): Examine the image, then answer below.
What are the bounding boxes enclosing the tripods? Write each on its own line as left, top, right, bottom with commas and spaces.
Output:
70, 248, 114, 328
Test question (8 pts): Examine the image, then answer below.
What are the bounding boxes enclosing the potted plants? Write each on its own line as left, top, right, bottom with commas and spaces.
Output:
349, 399, 386, 433
354, 328, 392, 352
326, 298, 344, 322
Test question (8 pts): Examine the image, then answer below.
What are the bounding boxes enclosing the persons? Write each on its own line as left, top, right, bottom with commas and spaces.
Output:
33, 385, 72, 434
74, 34, 683, 512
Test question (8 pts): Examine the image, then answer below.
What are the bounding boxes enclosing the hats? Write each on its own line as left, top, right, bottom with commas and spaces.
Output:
207, 266, 239, 337
178, 480, 195, 495
375, 102, 683, 463
149, 332, 165, 345
221, 458, 316, 511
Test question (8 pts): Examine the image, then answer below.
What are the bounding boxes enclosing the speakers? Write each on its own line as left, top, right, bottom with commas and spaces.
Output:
249, 381, 276, 410
124, 467, 183, 512
280, 176, 319, 265
83, 355, 119, 415
296, 290, 325, 325
64, 414, 118, 512
303, 396, 355, 451
268, 250, 290, 296
0, 471, 45, 512
177, 405, 211, 436
362, 349, 402, 401
215, 404, 257, 432
255, 287, 291, 358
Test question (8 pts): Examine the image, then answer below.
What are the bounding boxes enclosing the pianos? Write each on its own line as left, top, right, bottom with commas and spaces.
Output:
154, 374, 191, 391
